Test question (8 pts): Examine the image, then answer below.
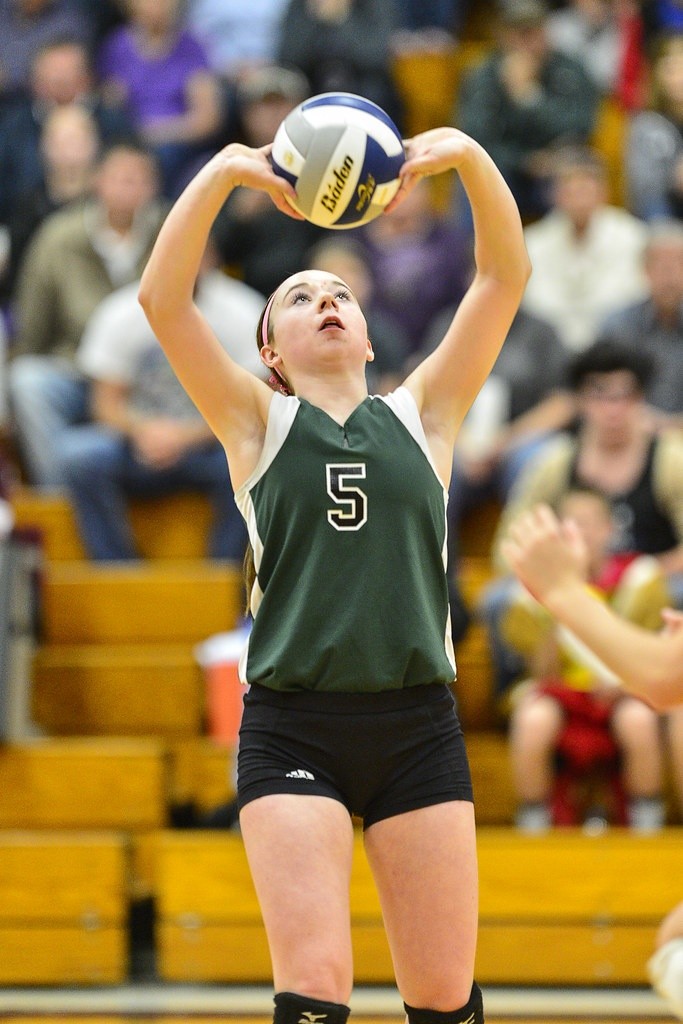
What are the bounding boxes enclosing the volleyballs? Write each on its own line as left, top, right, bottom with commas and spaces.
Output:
269, 90, 407, 232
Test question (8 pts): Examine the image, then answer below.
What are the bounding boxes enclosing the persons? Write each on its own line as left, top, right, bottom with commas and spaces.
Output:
648, 907, 683, 1018
1, 0, 683, 829
137, 126, 532, 1024
503, 503, 683, 712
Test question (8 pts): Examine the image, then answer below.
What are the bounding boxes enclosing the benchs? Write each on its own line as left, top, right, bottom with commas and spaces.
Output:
1, 36, 683, 985
